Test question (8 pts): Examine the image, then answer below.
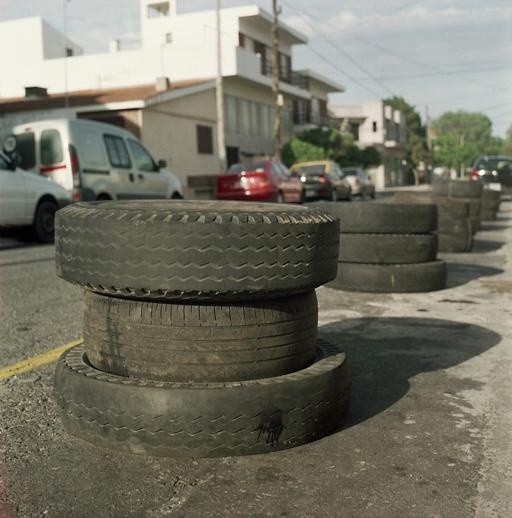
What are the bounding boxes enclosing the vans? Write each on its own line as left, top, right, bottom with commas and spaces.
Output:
4, 116, 184, 200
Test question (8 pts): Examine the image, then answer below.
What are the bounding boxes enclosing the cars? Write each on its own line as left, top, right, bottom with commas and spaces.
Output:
0, 150, 76, 242
289, 160, 352, 202
342, 166, 376, 199
470, 157, 512, 190
217, 157, 306, 202
